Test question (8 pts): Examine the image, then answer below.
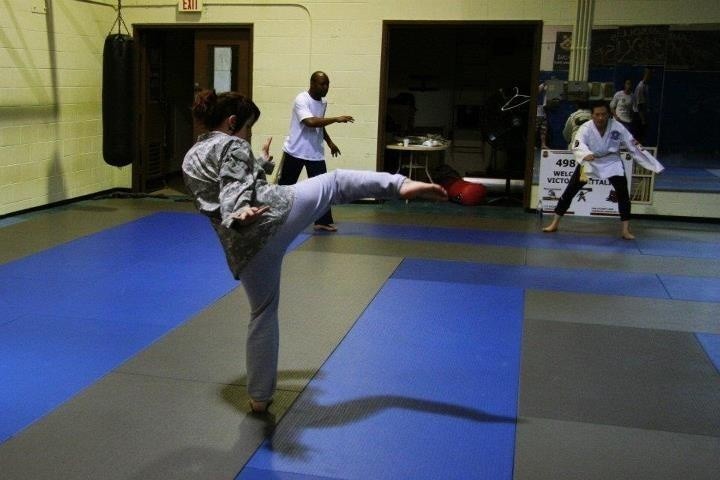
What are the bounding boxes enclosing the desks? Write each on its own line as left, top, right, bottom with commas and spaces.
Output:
385, 135, 451, 185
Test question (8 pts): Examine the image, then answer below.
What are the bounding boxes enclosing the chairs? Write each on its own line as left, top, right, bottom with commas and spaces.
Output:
445, 104, 492, 173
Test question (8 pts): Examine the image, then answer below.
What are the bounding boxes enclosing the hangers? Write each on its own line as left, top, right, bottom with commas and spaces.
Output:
495, 87, 532, 112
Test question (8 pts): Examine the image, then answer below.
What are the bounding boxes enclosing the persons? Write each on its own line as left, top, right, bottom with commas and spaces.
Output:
182, 88, 448, 411
275, 70, 356, 230
563, 104, 592, 150
542, 99, 665, 240
608, 69, 656, 142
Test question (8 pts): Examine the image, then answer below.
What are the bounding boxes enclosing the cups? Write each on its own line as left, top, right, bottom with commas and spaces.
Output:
403, 139, 410, 147
